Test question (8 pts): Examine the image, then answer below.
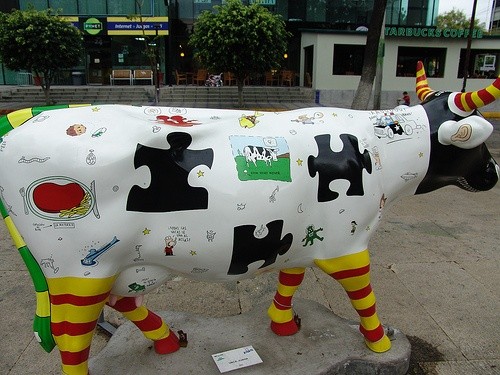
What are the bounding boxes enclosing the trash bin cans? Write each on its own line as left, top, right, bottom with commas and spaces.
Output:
71, 70, 85, 84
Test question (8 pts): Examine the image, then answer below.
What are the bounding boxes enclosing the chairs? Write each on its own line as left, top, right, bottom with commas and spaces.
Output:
265, 72, 278, 86
280, 70, 295, 86
224, 72, 237, 86
192, 69, 206, 85
175, 69, 187, 86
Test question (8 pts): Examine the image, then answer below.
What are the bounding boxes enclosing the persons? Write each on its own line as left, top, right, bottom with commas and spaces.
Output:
399, 91, 410, 106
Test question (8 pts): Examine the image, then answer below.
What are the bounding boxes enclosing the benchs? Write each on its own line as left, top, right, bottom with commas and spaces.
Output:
132, 70, 153, 85
110, 69, 131, 86
